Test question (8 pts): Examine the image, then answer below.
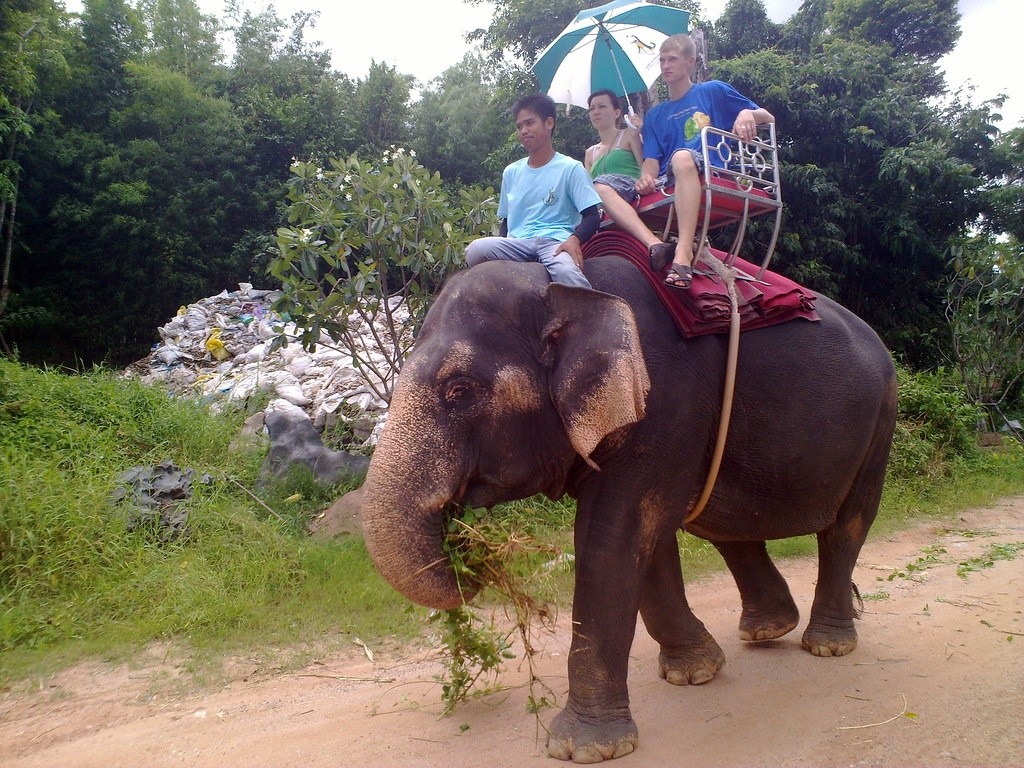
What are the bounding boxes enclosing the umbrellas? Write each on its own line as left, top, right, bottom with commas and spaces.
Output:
530, 0, 691, 131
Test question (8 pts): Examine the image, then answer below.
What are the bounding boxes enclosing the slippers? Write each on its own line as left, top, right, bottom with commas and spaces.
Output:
648, 241, 677, 272
663, 261, 693, 289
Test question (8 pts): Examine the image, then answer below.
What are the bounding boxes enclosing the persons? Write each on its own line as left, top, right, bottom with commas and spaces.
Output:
463, 95, 602, 291
594, 34, 774, 288
580, 88, 644, 182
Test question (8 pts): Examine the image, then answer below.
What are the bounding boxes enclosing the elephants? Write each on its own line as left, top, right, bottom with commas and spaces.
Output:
360, 241, 897, 763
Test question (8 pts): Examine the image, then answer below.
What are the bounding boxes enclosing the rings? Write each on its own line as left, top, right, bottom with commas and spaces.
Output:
741, 125, 746, 129
640, 184, 643, 188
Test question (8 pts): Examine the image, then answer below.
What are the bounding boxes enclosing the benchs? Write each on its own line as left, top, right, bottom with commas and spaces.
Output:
597, 120, 783, 286
